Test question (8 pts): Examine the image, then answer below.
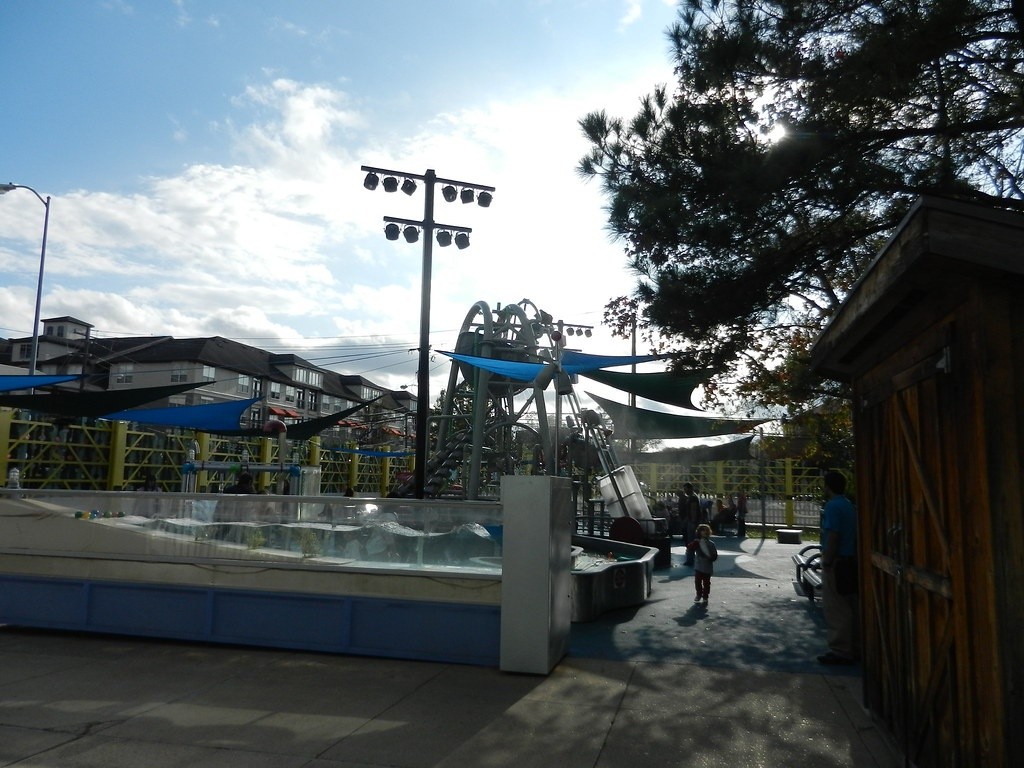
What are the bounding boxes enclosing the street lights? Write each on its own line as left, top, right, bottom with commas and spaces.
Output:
0, 184, 52, 488
358, 164, 498, 506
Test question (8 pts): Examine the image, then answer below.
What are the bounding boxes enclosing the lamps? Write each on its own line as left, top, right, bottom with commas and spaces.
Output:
566, 326, 574, 337
364, 171, 379, 190
576, 326, 583, 337
403, 224, 420, 244
585, 328, 592, 337
383, 220, 402, 241
400, 177, 417, 196
441, 183, 458, 202
436, 227, 454, 247
381, 174, 400, 193
476, 189, 494, 207
455, 230, 470, 250
460, 187, 474, 204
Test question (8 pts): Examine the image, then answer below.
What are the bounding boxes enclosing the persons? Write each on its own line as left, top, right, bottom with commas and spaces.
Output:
213, 472, 276, 523
686, 524, 717, 603
817, 468, 856, 662
649, 485, 747, 538
317, 488, 354, 541
131, 470, 162, 516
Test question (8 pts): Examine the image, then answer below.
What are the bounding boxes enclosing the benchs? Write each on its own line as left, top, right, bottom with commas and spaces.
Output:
791, 544, 823, 596
775, 529, 803, 544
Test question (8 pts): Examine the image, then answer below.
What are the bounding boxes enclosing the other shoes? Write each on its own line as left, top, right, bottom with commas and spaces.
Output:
817, 652, 841, 666
703, 596, 708, 603
684, 559, 693, 566
694, 595, 701, 601
